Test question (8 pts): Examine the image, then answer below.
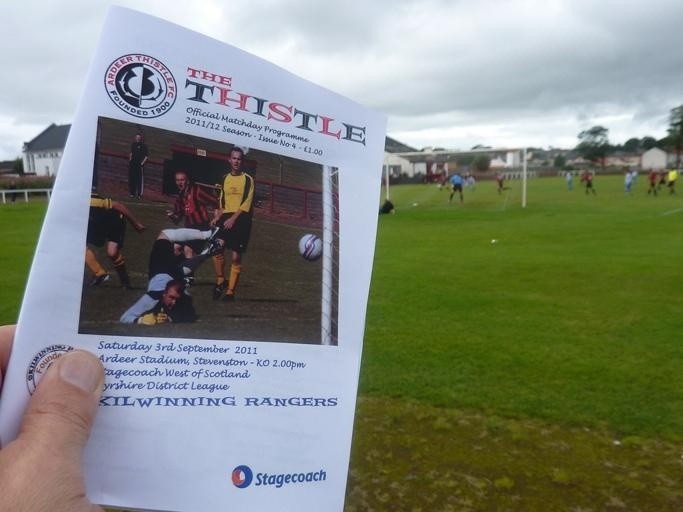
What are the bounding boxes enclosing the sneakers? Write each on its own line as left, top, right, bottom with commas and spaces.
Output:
88, 274, 111, 288
184, 282, 195, 288
213, 282, 235, 302
209, 225, 221, 254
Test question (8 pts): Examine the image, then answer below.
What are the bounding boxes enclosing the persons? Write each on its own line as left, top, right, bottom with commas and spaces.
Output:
85, 132, 255, 326
624, 168, 677, 195
495, 170, 510, 196
0, 325, 104, 512
566, 170, 597, 195
437, 170, 476, 204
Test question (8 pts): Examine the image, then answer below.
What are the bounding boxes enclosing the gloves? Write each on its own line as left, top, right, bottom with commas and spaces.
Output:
139, 312, 168, 325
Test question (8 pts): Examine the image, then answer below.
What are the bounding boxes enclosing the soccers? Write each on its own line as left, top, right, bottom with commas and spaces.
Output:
299, 234, 322, 260
492, 239, 496, 243
413, 203, 417, 206
